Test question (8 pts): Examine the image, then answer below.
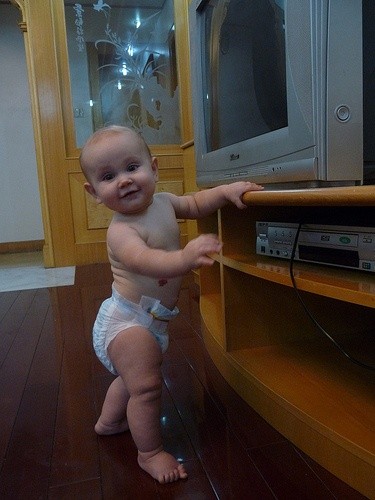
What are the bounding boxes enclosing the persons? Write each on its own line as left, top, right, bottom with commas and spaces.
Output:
79, 124, 265, 483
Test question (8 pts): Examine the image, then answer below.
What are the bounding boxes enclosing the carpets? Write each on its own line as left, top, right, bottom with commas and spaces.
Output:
0, 263, 77, 293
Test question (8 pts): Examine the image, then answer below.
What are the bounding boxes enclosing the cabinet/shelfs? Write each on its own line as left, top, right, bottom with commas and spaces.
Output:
195, 185, 375, 496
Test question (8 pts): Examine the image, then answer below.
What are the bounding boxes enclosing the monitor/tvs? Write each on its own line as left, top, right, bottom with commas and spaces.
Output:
190, 0, 374, 190
